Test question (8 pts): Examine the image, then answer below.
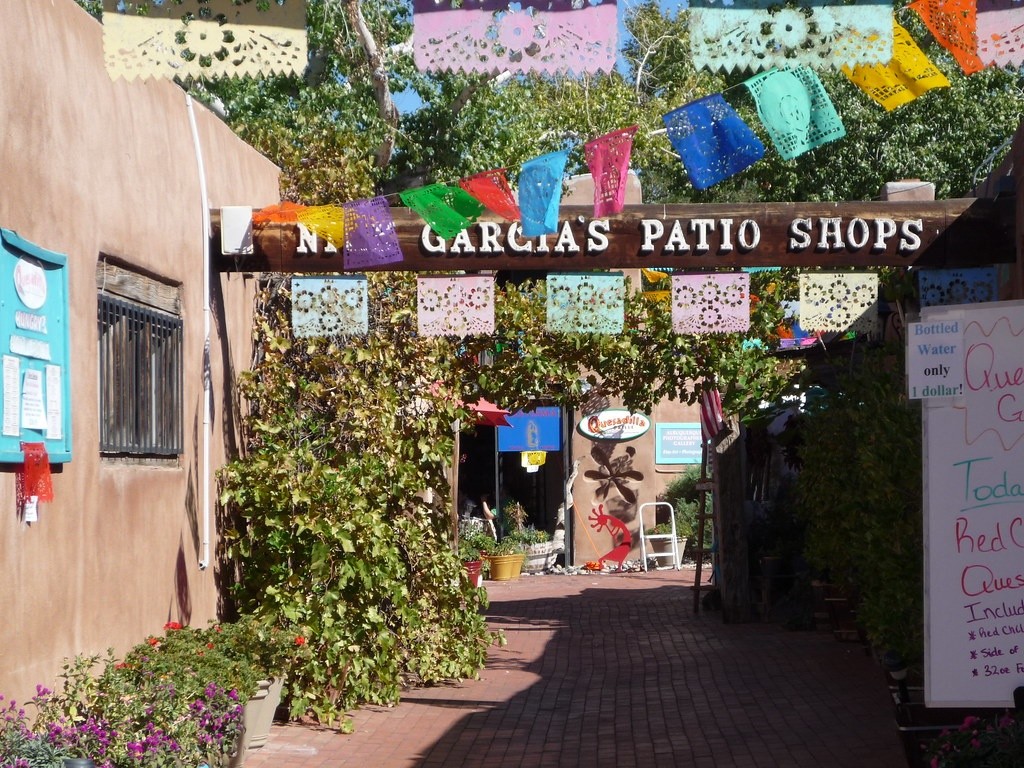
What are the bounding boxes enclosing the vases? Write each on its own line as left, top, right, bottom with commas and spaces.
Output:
252, 667, 286, 747
519, 540, 552, 573
216, 707, 249, 766
651, 534, 686, 567
235, 689, 267, 754
513, 550, 526, 577
479, 548, 491, 563
488, 552, 514, 580
464, 560, 483, 586
62, 755, 95, 767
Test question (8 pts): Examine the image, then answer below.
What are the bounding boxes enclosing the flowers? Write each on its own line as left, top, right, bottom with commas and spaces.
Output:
455, 529, 551, 561
919, 717, 1023, 768
1, 612, 304, 767
646, 516, 693, 539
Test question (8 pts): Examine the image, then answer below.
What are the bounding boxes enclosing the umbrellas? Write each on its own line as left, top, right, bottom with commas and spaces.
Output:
433, 379, 515, 429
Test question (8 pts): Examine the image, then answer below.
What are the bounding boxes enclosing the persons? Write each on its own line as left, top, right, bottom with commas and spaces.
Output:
459, 472, 498, 542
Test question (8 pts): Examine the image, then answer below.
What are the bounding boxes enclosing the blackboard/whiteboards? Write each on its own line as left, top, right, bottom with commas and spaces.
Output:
919, 299, 1024, 708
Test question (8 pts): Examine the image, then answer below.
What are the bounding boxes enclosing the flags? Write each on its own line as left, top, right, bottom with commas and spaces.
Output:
701, 378, 724, 442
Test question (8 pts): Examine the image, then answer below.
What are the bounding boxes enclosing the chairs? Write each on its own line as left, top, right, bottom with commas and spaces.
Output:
894, 661, 1023, 767
